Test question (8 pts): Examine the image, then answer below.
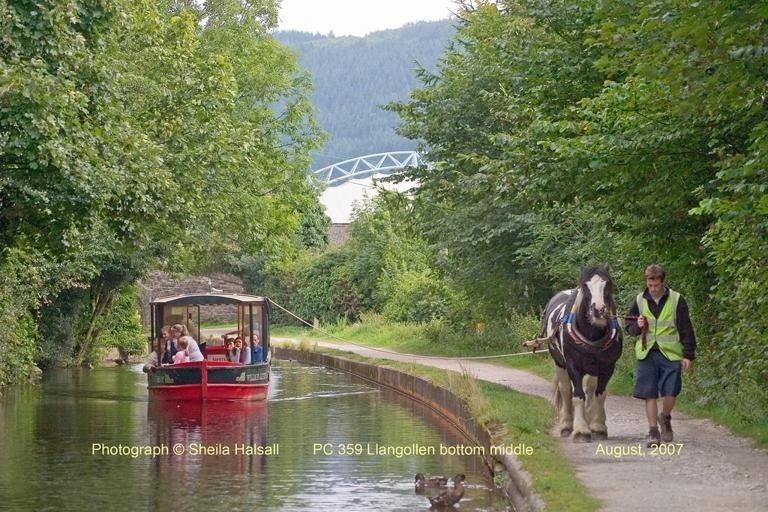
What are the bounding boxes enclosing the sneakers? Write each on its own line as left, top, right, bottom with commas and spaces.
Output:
658, 412, 674, 442
646, 427, 661, 449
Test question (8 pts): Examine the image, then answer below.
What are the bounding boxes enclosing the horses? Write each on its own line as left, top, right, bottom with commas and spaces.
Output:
539, 261, 626, 444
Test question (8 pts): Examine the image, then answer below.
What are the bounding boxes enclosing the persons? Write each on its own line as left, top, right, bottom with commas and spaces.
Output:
625, 265, 698, 448
143, 318, 263, 373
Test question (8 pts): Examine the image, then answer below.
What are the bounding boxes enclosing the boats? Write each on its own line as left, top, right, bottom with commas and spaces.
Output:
144, 294, 269, 404
